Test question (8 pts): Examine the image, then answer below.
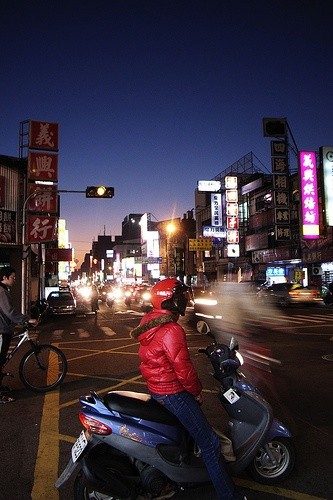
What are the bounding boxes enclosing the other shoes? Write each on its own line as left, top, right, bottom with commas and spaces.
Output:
0, 395, 13, 402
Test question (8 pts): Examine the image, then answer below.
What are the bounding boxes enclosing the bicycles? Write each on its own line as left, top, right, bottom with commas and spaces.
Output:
0, 323, 68, 394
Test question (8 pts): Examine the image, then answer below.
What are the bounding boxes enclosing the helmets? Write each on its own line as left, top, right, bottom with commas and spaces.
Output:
151, 279, 188, 313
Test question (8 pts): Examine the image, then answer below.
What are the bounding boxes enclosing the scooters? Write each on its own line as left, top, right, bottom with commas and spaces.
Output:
54, 320, 295, 500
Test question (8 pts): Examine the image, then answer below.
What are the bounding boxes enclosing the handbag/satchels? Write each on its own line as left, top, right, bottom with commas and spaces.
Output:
193, 427, 236, 462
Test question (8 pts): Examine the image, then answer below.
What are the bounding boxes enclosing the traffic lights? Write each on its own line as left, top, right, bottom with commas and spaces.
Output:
85, 186, 115, 198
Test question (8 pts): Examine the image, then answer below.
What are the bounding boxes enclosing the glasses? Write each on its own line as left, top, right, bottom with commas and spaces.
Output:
8, 276, 16, 280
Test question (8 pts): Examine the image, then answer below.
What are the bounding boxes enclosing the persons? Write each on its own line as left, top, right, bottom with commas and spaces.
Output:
73, 281, 136, 309
0, 266, 38, 404
130, 279, 247, 500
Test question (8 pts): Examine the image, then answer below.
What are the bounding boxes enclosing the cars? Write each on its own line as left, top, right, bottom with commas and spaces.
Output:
255, 282, 322, 307
45, 280, 195, 321
